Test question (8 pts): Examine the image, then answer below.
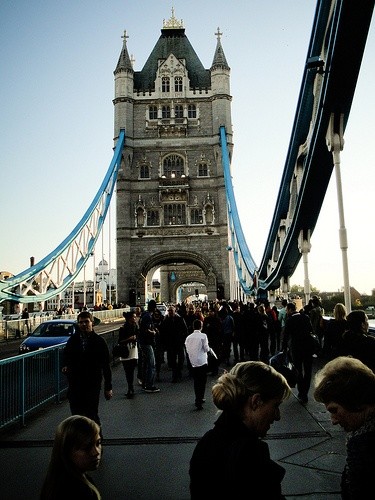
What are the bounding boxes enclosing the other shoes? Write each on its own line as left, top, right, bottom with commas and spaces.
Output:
298, 393, 308, 403
127, 392, 136, 399
144, 386, 161, 392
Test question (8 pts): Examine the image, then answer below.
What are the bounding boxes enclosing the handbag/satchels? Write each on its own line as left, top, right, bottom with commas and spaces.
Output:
301, 312, 320, 353
111, 340, 130, 358
120, 341, 139, 360
268, 349, 299, 388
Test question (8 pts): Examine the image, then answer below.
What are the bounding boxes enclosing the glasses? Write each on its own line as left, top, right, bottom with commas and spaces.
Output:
78, 321, 92, 325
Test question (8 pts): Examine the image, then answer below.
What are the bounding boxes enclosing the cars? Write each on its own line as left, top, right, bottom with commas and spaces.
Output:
156, 305, 169, 316
19, 319, 77, 366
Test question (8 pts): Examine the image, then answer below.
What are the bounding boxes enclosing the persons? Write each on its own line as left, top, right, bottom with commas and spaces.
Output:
118, 297, 375, 411
36, 415, 104, 500
312, 356, 375, 500
185, 362, 290, 499
59, 311, 114, 441
18, 307, 30, 332
56, 302, 124, 317
